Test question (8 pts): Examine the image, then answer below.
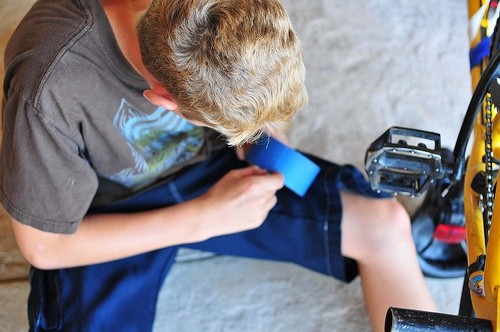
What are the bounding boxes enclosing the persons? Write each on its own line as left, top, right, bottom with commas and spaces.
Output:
0, 1, 437, 332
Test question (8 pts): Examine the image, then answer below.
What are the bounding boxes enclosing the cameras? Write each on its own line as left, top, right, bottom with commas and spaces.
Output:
365, 127, 443, 200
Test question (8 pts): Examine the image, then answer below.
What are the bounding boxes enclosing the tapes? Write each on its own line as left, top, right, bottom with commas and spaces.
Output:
244, 131, 321, 199
